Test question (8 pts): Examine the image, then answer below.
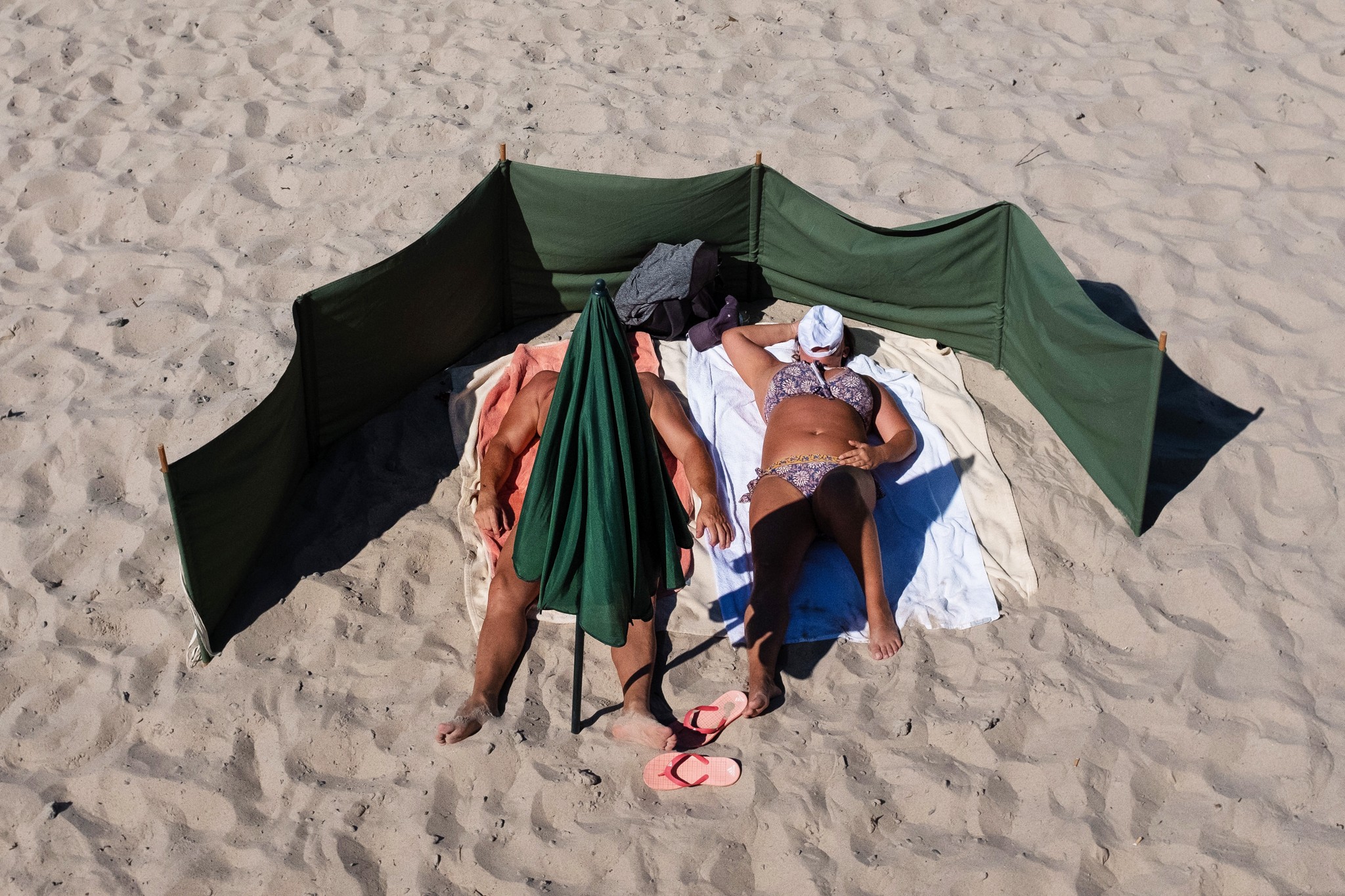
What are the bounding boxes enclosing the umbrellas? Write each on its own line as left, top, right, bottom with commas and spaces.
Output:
511, 279, 696, 734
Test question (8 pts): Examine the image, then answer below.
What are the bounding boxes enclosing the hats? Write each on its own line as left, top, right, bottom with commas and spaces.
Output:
798, 305, 844, 358
688, 295, 738, 353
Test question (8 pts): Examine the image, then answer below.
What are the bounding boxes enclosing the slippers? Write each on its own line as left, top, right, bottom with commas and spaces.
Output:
643, 752, 740, 791
667, 690, 748, 748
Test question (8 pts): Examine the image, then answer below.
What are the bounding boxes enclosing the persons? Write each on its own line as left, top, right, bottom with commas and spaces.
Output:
722, 319, 917, 719
438, 319, 736, 744
